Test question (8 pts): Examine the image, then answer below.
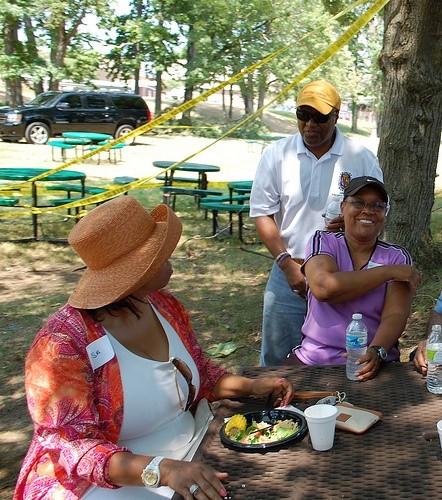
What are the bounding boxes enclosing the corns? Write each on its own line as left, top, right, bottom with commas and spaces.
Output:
225, 414, 246, 439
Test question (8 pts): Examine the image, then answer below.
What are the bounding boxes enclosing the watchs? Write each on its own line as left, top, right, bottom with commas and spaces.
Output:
142, 455, 165, 488
369, 345, 388, 369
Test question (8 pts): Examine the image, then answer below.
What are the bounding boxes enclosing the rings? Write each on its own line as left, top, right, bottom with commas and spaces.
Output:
190, 484, 200, 495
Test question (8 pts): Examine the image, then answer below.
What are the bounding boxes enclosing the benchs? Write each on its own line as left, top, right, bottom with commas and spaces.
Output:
156, 176, 250, 244
0, 176, 140, 225
46, 139, 126, 165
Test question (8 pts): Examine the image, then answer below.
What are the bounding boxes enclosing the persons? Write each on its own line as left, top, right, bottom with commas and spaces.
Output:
292, 177, 442, 382
12, 196, 295, 500
249, 79, 390, 366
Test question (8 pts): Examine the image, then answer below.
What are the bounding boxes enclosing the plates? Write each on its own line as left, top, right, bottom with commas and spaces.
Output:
219, 409, 307, 449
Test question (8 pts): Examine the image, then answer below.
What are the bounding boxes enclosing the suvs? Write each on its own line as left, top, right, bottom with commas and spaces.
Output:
0, 91, 151, 145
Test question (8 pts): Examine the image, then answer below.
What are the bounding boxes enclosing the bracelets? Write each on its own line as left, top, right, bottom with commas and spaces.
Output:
276, 252, 291, 268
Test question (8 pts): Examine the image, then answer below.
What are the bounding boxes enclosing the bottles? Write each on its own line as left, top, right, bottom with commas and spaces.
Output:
426, 324, 442, 394
324, 195, 341, 232
345, 313, 368, 381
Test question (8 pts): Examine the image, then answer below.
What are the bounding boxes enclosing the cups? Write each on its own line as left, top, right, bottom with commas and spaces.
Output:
437, 420, 442, 450
304, 404, 337, 451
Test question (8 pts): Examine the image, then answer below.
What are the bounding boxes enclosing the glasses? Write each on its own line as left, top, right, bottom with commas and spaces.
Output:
296, 108, 337, 124
169, 356, 197, 412
345, 199, 385, 210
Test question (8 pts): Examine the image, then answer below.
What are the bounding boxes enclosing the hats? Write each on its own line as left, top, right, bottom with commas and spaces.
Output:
345, 176, 389, 203
67, 195, 183, 310
296, 80, 341, 115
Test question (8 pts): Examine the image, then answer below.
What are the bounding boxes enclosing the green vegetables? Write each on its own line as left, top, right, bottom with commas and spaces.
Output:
231, 419, 299, 444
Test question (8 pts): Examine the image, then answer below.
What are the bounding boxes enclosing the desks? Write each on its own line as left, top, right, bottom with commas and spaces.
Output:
227, 178, 255, 233
0, 168, 86, 245
153, 161, 221, 208
61, 132, 112, 164
167, 363, 442, 500
